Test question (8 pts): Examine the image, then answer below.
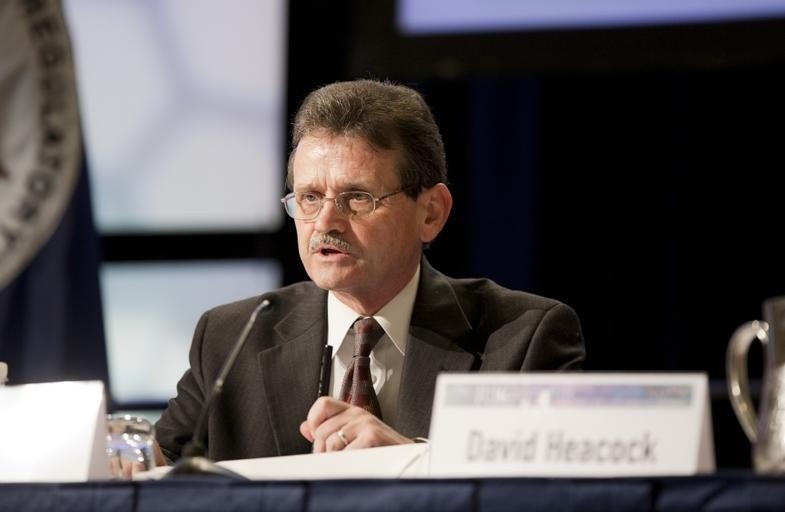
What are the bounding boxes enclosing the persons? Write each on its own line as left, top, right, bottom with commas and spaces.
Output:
109, 81, 590, 480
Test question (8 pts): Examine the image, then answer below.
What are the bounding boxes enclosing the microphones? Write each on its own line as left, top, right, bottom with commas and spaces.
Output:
158, 290, 282, 484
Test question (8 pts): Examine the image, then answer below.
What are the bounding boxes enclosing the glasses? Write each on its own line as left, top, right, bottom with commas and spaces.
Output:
280, 189, 403, 222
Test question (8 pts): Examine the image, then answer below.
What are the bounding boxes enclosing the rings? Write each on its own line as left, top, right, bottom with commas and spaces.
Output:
336, 429, 348, 447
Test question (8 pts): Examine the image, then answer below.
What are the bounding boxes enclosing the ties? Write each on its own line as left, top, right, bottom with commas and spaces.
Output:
337, 316, 385, 422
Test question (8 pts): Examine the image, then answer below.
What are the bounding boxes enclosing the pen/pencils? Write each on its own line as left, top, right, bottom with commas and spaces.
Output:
318, 345, 333, 397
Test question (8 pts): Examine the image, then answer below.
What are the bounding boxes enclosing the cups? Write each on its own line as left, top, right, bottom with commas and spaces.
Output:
724, 296, 785, 479
